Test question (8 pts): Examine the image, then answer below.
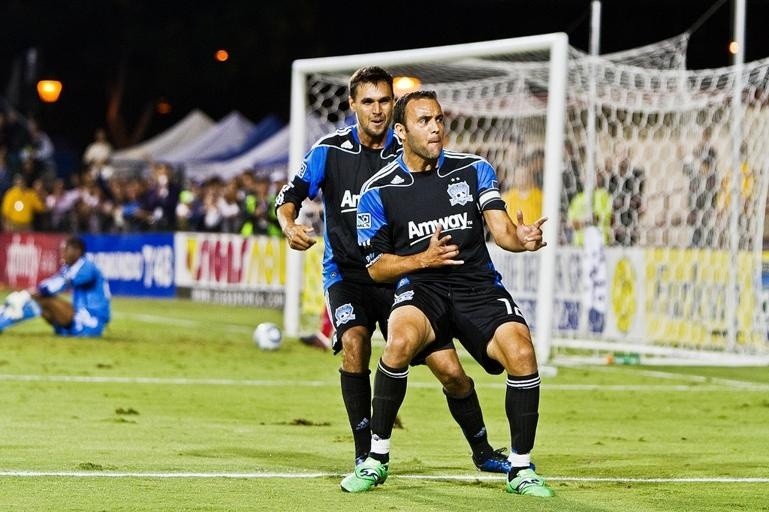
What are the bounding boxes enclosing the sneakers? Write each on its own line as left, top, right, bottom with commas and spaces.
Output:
341, 455, 388, 493
505, 465, 556, 499
472, 450, 534, 473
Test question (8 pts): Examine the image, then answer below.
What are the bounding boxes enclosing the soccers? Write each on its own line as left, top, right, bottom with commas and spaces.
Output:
254, 322, 283, 350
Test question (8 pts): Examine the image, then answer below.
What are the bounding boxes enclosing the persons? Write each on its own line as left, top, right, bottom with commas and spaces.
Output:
2, 237, 111, 338
273, 63, 536, 475
487, 131, 768, 246
339, 87, 555, 501
1, 108, 324, 237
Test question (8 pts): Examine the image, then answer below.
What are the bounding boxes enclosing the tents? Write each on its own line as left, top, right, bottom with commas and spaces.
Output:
161, 111, 256, 167
206, 116, 287, 166
194, 114, 337, 184
112, 110, 217, 168
